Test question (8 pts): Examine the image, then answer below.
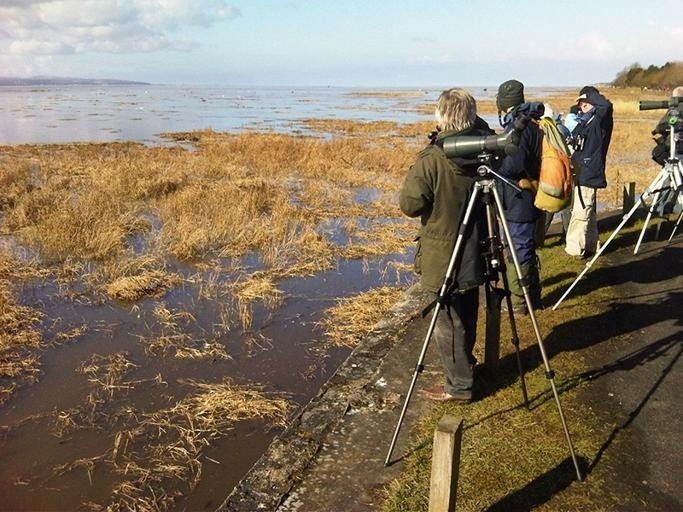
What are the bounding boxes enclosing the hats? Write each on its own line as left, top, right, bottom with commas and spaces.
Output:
495, 79, 525, 112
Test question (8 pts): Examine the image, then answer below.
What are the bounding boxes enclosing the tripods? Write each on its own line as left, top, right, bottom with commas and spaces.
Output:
382, 179, 587, 485
551, 155, 683, 311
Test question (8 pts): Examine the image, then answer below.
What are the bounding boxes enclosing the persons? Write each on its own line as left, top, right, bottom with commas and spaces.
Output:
496, 80, 543, 313
652, 86, 683, 213
564, 87, 613, 260
543, 103, 579, 233
399, 88, 486, 402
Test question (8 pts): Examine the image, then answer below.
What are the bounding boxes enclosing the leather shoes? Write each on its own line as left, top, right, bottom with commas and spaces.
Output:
420, 384, 472, 404
557, 251, 584, 260
484, 306, 527, 315
